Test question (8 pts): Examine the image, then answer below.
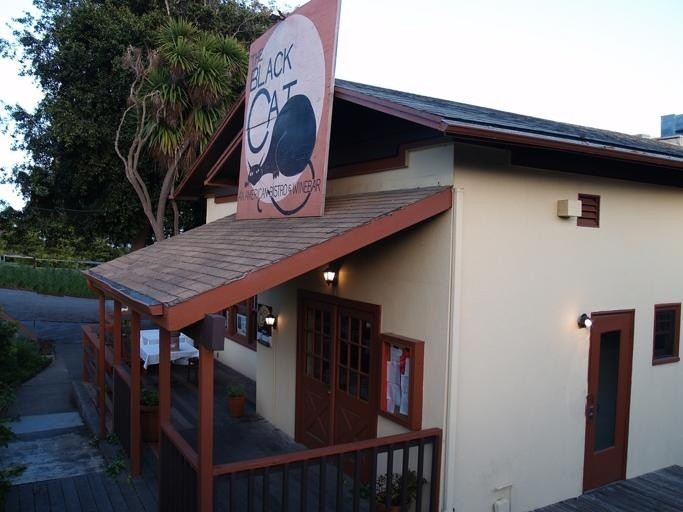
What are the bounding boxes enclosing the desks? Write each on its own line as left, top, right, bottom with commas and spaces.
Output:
140, 330, 199, 391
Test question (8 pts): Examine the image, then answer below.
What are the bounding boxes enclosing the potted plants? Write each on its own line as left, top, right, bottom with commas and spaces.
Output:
343, 469, 428, 512
140, 388, 173, 443
225, 383, 245, 417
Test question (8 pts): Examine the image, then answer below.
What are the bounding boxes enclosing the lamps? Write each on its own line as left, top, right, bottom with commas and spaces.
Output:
265, 314, 277, 330
323, 265, 337, 287
577, 313, 592, 328
